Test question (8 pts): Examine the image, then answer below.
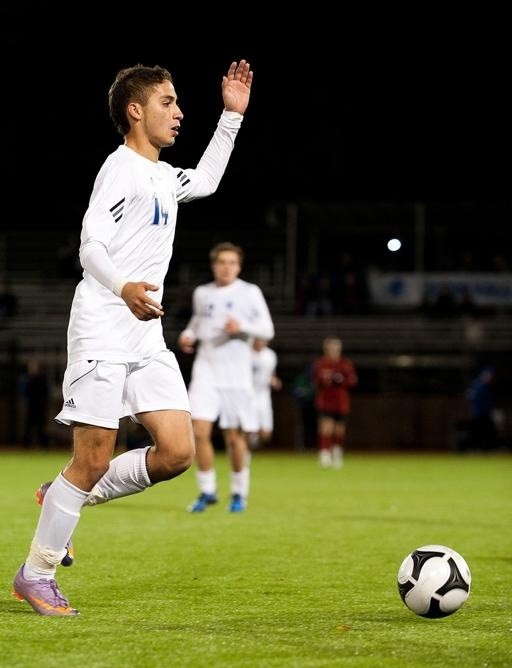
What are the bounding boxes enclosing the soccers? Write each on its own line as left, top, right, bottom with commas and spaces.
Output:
397, 544, 471, 618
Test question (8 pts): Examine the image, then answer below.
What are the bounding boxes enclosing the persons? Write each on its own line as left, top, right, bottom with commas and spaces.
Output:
13, 58, 255, 619
313, 251, 510, 319
309, 335, 357, 470
17, 359, 50, 448
468, 369, 503, 452
245, 338, 282, 450
177, 242, 275, 514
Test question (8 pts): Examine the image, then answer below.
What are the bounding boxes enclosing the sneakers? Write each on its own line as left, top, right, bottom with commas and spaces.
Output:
11, 562, 80, 616
34, 480, 74, 566
186, 492, 219, 513
229, 492, 246, 512
